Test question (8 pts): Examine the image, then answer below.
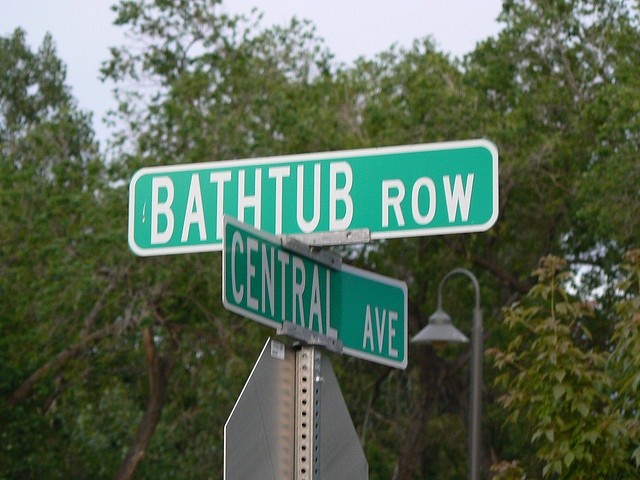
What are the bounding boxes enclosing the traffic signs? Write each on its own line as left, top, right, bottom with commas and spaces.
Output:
128, 139, 499, 258
221, 212, 407, 372
219, 333, 371, 479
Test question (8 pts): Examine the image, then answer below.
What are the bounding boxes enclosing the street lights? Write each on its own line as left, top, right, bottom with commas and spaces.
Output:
410, 268, 487, 480
